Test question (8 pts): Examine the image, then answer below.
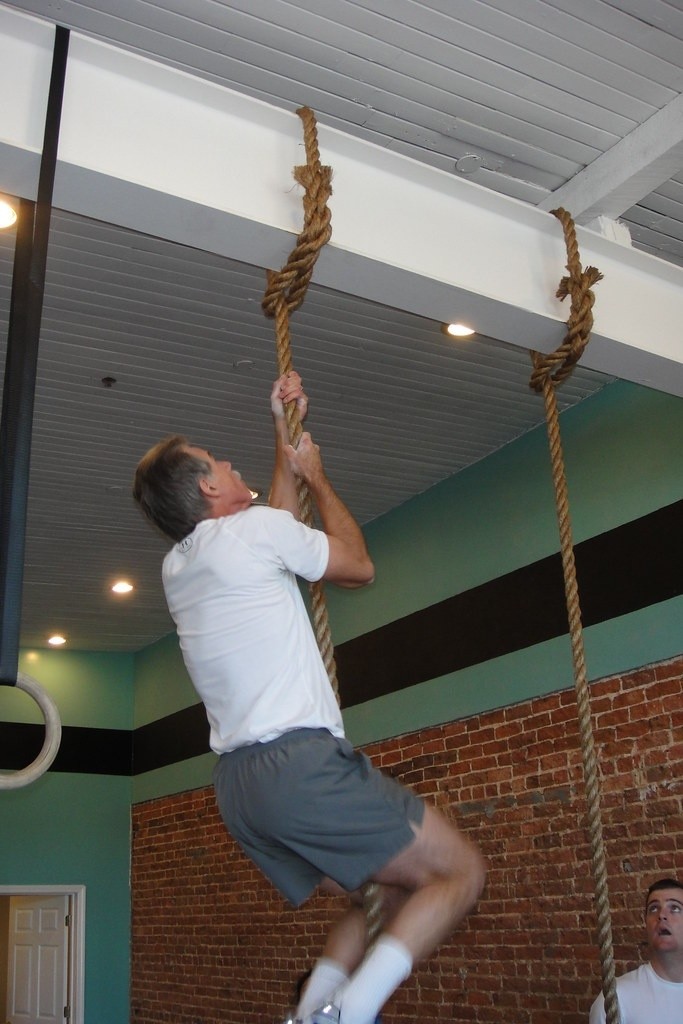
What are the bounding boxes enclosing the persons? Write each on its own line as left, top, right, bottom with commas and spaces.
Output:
588, 877, 683, 1024
130, 369, 487, 1024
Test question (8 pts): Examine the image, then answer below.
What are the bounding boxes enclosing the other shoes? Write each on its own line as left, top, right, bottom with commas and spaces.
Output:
284, 1016, 302, 1024
310, 1002, 340, 1024
